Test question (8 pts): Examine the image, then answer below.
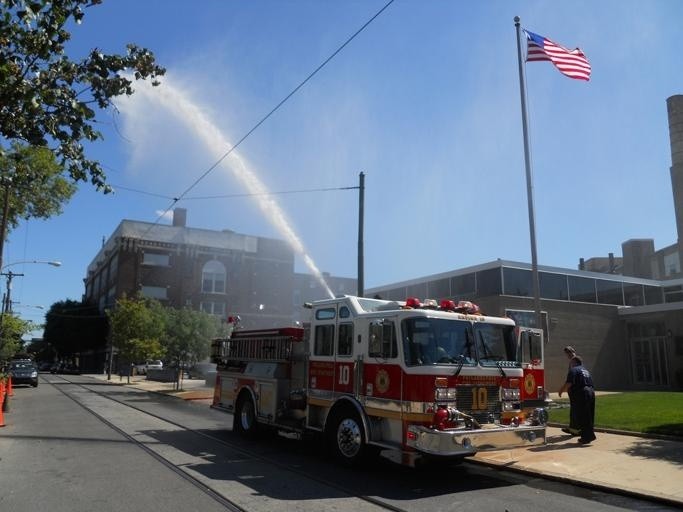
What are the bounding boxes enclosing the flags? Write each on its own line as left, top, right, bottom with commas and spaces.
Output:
521, 28, 591, 82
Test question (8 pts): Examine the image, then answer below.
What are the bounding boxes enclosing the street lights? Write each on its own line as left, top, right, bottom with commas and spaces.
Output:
13, 304, 44, 311
1, 260, 62, 270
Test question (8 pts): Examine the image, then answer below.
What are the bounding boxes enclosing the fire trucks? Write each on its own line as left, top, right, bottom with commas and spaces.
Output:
209, 296, 552, 468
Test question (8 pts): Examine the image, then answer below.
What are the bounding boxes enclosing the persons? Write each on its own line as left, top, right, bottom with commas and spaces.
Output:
559, 356, 597, 446
559, 345, 581, 436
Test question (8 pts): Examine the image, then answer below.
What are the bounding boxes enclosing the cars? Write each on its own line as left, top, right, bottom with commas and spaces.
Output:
188, 362, 217, 379
6, 359, 38, 387
134, 359, 163, 375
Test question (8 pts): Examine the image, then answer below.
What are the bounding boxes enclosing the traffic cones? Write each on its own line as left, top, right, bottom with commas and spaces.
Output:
0, 376, 13, 427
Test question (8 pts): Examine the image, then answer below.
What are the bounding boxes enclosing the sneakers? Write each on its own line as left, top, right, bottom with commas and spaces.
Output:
579, 434, 596, 444
562, 428, 578, 437
